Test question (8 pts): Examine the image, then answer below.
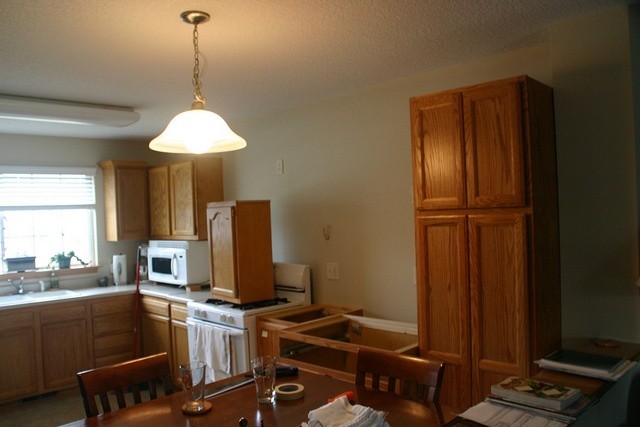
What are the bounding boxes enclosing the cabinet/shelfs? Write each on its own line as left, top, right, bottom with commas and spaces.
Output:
149, 157, 225, 241
98, 159, 152, 242
204, 198, 275, 307
139, 298, 192, 385
408, 72, 563, 413
91, 292, 137, 366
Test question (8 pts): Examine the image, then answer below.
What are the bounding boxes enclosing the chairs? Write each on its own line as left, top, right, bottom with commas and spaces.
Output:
74, 350, 184, 412
356, 346, 448, 412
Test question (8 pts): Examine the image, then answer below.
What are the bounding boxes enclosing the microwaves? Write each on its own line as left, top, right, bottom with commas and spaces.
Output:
147, 238, 213, 287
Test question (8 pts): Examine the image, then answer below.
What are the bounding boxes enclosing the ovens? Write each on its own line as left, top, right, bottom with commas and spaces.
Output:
184, 317, 250, 387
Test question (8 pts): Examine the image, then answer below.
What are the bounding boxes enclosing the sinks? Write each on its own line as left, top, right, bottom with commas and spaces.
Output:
0, 292, 29, 308
31, 289, 76, 303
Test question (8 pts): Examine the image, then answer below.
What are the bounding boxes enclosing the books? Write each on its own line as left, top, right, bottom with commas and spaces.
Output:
538, 349, 637, 381
452, 397, 577, 427
492, 376, 582, 410
486, 398, 587, 422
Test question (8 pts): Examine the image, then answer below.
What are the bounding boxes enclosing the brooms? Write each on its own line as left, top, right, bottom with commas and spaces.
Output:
111, 247, 149, 391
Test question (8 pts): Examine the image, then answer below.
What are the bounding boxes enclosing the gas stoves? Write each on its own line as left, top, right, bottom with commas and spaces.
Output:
187, 295, 297, 315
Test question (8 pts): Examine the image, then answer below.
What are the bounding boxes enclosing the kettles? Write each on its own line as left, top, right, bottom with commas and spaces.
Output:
112, 253, 129, 287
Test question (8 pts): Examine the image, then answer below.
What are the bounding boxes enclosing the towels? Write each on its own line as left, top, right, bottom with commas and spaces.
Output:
309, 396, 391, 427
192, 323, 233, 383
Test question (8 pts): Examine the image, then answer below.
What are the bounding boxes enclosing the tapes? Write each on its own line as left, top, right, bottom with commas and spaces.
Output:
275, 383, 305, 401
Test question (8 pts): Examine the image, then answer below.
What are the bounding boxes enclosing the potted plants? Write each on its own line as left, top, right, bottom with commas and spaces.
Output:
47, 251, 87, 269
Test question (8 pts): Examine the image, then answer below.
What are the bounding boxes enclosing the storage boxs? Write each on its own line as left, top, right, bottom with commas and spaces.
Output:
0, 308, 93, 401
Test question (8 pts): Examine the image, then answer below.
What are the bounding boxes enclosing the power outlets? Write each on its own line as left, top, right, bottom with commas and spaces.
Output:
326, 262, 340, 281
273, 160, 287, 176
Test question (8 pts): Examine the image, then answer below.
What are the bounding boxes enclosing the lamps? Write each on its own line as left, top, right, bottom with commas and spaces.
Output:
147, 13, 250, 156
0, 92, 144, 130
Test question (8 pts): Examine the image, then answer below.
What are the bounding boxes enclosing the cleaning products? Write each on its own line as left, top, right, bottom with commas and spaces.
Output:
48, 266, 62, 289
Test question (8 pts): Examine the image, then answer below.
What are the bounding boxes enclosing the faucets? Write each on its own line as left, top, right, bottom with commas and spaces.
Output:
8, 276, 24, 293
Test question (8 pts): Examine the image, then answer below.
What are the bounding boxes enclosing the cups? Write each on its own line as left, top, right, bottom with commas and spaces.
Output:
250, 354, 279, 405
177, 361, 208, 411
